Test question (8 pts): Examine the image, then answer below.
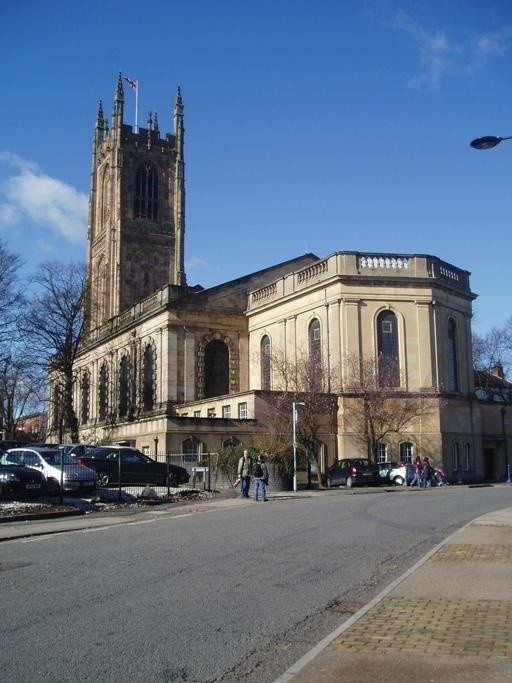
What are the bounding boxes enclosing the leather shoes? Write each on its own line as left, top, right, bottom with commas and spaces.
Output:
264, 499, 268, 501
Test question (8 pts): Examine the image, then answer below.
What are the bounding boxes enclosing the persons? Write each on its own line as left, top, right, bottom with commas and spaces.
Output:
410, 456, 423, 486
421, 457, 434, 488
252, 454, 268, 502
237, 449, 254, 498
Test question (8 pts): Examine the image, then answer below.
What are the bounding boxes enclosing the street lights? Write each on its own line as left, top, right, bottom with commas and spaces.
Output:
293, 401, 306, 492
470, 135, 512, 150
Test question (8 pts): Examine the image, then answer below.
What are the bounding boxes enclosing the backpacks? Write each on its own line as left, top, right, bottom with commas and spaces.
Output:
253, 461, 263, 477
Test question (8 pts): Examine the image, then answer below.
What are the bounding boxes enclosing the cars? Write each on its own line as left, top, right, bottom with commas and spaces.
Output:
327, 458, 416, 487
0, 443, 190, 497
